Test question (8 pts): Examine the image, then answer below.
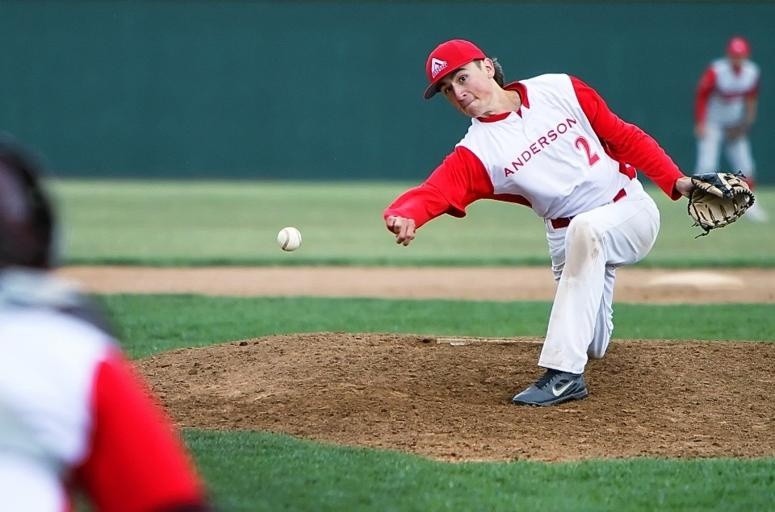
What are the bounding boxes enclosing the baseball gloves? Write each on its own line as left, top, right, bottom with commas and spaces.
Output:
687, 170, 755, 239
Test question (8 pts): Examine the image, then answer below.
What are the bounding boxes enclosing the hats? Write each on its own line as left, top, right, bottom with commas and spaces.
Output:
422, 39, 487, 100
727, 35, 752, 58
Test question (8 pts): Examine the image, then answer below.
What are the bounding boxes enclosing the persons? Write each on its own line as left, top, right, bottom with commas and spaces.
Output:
693, 37, 768, 221
382, 38, 696, 407
0, 136, 208, 511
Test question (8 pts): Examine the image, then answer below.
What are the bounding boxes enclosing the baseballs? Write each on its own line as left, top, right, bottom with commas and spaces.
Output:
277, 227, 302, 251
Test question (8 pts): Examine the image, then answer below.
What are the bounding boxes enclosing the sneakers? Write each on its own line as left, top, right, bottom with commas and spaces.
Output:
512, 369, 588, 407
